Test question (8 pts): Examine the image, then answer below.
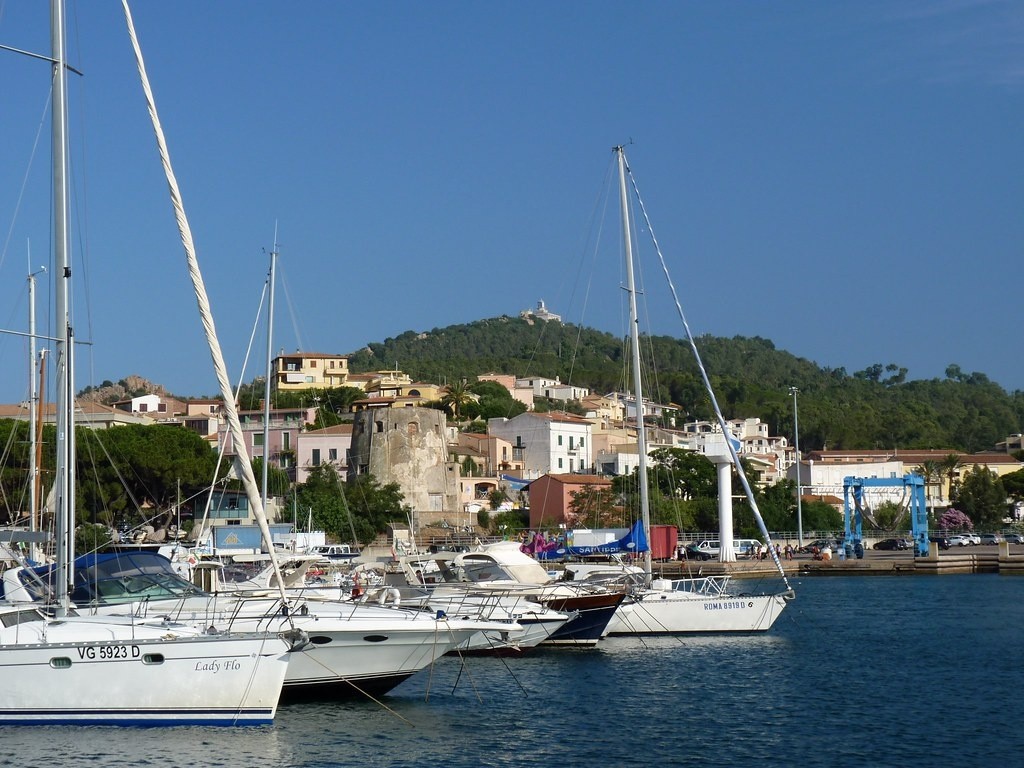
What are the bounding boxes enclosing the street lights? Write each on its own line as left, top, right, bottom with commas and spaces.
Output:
786, 383, 805, 551
465, 484, 474, 545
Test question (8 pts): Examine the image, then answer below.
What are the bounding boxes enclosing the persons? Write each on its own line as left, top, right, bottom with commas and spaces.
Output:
757, 546, 761, 559
750, 545, 756, 559
745, 545, 750, 559
350, 571, 364, 598
768, 543, 799, 560
812, 545, 845, 561
671, 546, 678, 561
678, 545, 686, 560
416, 569, 422, 583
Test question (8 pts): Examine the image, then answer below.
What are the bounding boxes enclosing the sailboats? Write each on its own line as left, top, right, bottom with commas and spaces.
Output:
0, 2, 652, 725
499, 144, 798, 637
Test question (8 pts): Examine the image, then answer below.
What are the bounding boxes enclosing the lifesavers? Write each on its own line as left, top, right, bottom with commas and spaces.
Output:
379, 587, 401, 610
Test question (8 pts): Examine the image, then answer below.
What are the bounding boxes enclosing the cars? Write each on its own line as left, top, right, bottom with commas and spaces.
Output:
873, 538, 904, 551
929, 536, 952, 551
801, 539, 846, 554
959, 534, 981, 546
979, 533, 1001, 545
947, 536, 970, 547
1002, 533, 1024, 545
900, 538, 914, 550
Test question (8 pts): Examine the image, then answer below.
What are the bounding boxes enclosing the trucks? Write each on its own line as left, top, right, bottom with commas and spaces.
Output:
688, 538, 763, 560
